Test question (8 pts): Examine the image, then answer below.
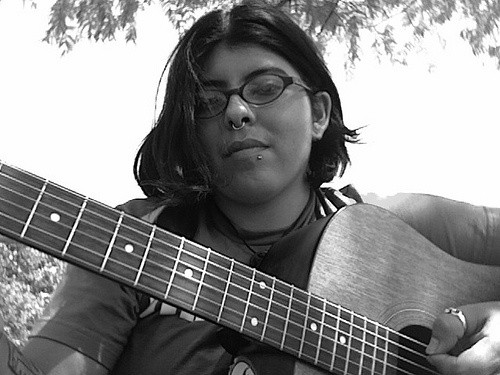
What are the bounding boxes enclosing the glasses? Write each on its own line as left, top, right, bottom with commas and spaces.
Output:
191, 73, 314, 120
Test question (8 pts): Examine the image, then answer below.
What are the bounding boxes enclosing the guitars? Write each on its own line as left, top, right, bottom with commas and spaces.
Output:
0, 159, 500, 374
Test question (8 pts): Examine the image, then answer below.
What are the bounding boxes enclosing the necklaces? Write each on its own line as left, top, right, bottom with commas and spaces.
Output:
210, 177, 314, 257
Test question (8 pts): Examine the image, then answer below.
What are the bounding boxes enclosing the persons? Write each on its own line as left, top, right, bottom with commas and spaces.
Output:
1, 3, 500, 375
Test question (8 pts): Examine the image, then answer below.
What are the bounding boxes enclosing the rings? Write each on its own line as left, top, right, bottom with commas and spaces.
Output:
445, 307, 467, 334
